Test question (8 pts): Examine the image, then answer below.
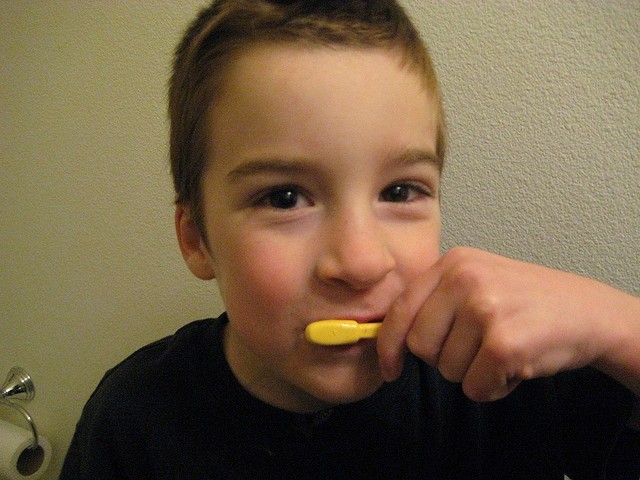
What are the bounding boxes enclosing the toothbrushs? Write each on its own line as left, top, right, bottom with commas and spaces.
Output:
305, 319, 383, 346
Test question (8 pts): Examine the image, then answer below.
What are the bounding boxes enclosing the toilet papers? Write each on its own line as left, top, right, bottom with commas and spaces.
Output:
0, 418, 52, 480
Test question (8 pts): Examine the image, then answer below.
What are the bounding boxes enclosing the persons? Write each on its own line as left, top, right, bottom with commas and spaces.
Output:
58, 0, 639, 480
